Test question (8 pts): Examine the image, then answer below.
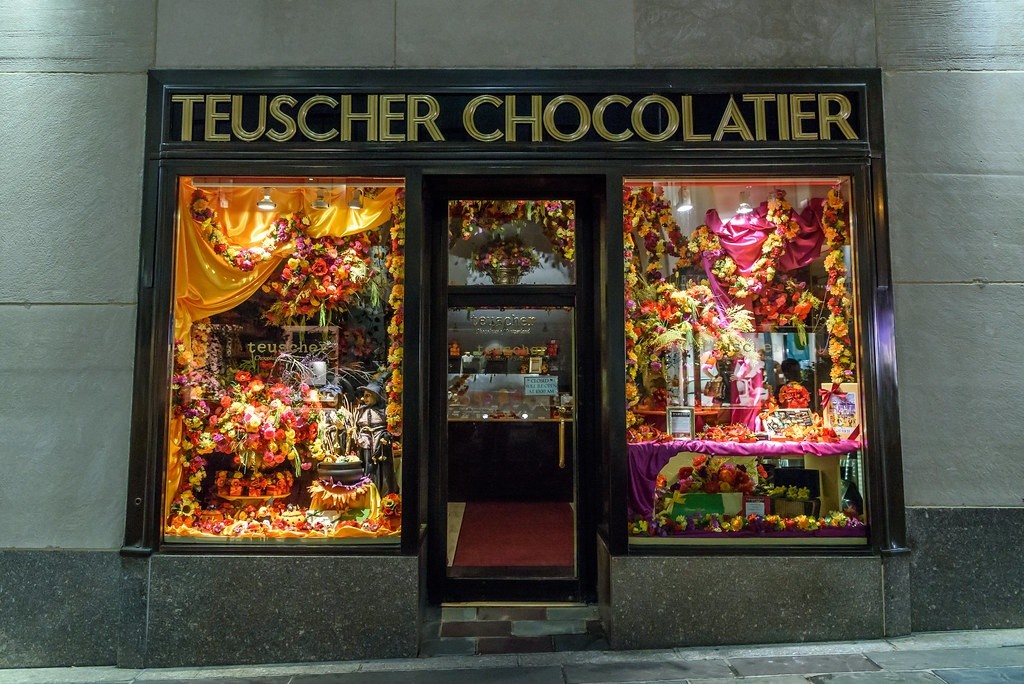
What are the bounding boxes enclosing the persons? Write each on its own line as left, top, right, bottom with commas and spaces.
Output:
353, 371, 399, 496
775, 358, 815, 412
758, 349, 781, 396
324, 392, 352, 422
704, 348, 750, 408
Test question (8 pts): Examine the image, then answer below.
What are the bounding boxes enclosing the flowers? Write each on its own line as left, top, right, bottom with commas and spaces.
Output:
171, 184, 862, 535
